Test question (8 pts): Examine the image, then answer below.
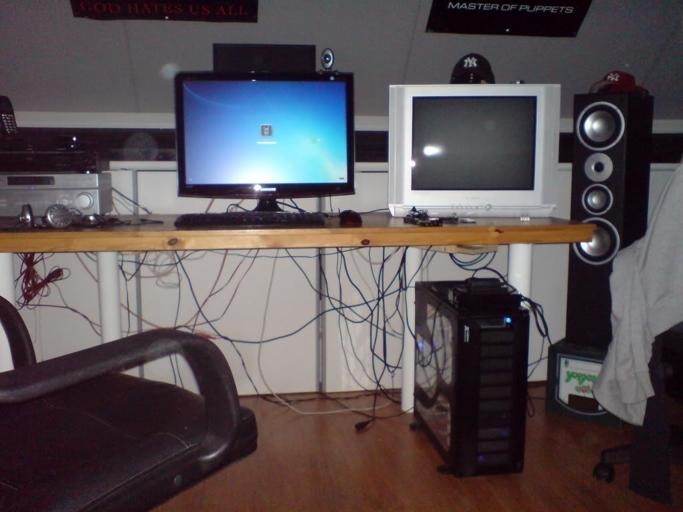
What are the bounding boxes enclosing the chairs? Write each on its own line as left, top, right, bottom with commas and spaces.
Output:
1, 295, 260, 511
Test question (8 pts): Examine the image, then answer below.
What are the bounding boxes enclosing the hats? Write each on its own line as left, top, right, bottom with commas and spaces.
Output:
601, 69, 650, 93
449, 52, 497, 84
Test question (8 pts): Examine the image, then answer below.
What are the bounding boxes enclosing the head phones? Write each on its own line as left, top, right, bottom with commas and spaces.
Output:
17, 203, 75, 230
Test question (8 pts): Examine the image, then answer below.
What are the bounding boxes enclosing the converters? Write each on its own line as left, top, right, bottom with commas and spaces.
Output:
465, 277, 501, 287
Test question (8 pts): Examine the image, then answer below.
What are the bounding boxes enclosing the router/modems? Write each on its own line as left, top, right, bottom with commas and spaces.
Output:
448, 288, 522, 310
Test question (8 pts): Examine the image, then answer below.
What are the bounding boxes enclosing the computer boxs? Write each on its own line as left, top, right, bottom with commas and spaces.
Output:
415, 281, 531, 479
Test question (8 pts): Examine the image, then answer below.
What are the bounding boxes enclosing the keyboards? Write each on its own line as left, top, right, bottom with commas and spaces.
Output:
174, 210, 327, 228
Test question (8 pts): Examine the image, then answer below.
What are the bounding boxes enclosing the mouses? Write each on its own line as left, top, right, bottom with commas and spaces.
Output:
339, 210, 362, 227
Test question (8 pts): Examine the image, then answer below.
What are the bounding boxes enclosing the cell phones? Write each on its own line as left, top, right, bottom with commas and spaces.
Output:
0, 94, 18, 135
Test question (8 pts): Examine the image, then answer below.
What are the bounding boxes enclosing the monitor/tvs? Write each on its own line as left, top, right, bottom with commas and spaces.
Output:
387, 83, 561, 220
172, 71, 355, 211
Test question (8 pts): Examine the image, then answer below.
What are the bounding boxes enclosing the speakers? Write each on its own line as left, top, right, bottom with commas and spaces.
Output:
561, 94, 656, 358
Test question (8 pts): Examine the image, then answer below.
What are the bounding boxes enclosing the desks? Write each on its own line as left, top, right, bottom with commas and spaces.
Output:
0, 212, 598, 415
